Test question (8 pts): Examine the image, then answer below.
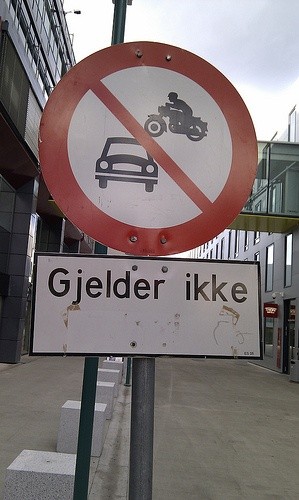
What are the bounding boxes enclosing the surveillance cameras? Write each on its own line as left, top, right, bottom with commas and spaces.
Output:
272, 293, 277, 299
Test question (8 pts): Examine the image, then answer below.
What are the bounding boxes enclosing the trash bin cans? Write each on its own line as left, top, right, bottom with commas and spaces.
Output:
288, 358, 299, 383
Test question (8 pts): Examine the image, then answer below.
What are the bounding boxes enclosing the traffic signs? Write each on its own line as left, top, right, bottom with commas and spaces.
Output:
30, 252, 265, 358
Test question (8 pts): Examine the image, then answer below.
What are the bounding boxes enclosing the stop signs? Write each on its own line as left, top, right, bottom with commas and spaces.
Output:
23, 41, 259, 258
